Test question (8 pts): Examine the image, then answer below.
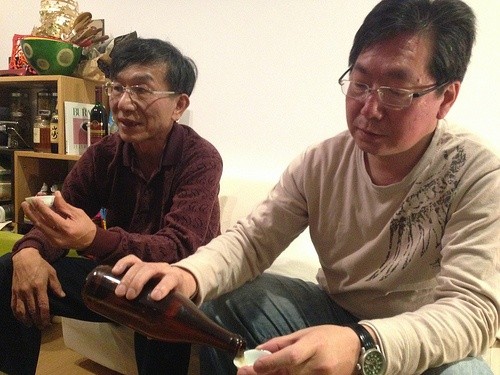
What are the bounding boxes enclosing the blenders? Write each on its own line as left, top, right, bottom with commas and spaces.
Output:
36, 89, 57, 118
6, 90, 31, 147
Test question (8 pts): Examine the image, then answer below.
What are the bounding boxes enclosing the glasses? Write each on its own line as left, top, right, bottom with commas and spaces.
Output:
337, 63, 452, 111
100, 79, 186, 102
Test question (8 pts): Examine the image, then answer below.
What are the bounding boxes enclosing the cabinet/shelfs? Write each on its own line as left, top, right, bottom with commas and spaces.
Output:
1, 74, 96, 233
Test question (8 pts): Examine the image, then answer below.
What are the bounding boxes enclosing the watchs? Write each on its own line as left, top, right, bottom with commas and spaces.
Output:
349, 324, 386, 375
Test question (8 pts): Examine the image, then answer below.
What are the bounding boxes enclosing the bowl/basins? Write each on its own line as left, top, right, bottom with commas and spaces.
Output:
20, 37, 81, 74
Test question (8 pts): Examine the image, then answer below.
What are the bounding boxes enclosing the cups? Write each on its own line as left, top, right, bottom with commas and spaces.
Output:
25, 196, 56, 209
233, 349, 273, 369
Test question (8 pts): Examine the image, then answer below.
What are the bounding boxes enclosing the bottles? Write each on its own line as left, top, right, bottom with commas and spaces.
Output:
34, 110, 60, 153
90, 86, 110, 146
81, 265, 249, 356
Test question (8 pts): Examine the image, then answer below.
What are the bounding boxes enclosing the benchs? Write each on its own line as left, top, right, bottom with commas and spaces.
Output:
58, 181, 500, 375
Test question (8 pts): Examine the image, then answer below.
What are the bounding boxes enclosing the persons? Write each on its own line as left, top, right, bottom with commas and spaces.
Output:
0, 38, 224, 375
112, 0, 500, 375
